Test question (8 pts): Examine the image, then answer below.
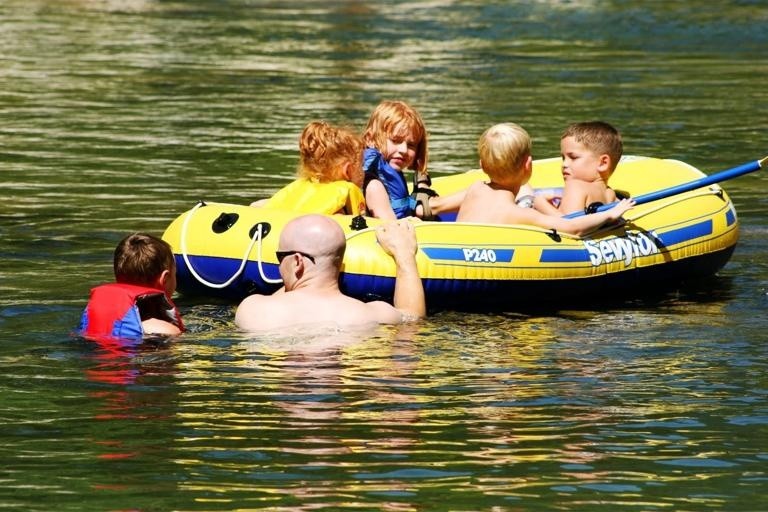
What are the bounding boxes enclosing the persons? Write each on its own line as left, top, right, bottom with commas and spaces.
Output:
357, 99, 430, 219
247, 121, 366, 218
67, 231, 185, 337
531, 121, 625, 217
233, 213, 426, 333
417, 121, 637, 237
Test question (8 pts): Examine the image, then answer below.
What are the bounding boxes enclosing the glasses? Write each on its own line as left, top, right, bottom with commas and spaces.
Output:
276, 251, 315, 264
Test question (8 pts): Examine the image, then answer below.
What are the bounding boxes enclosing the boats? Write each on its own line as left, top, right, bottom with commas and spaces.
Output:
162, 153, 739, 311
